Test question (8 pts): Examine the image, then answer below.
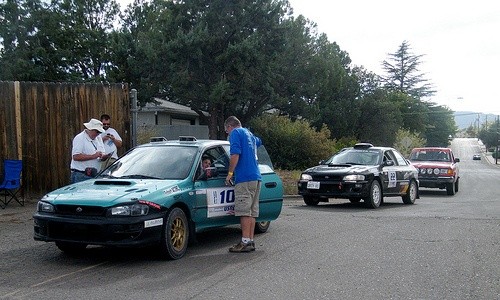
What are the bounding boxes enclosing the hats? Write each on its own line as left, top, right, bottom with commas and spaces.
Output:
83, 118, 105, 132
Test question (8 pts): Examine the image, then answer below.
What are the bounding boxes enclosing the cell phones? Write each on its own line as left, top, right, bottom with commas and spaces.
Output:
107, 133, 110, 135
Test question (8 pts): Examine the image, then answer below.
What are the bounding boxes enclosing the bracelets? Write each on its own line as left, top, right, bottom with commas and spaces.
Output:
227, 171, 234, 177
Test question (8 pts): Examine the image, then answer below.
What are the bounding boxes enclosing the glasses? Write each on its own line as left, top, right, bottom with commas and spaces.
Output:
225, 126, 229, 133
103, 124, 110, 125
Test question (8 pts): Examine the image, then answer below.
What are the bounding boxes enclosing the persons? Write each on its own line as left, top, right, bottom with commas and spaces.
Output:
222, 117, 263, 254
200, 156, 213, 178
69, 117, 113, 184
97, 114, 123, 174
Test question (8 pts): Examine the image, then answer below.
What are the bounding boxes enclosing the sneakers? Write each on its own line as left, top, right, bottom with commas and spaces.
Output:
233, 243, 255, 251
229, 241, 251, 252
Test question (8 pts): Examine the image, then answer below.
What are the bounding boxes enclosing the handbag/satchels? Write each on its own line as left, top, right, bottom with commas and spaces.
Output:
101, 157, 116, 171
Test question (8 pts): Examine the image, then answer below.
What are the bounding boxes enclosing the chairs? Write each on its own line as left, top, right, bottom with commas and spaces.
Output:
0, 160, 25, 209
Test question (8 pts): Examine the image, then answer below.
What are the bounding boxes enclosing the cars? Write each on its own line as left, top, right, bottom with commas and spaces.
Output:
31, 133, 286, 259
403, 147, 460, 195
297, 141, 419, 209
473, 154, 481, 160
